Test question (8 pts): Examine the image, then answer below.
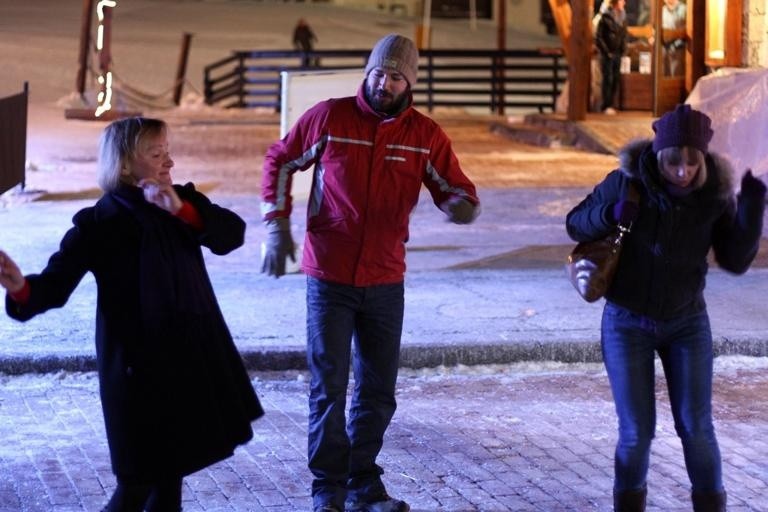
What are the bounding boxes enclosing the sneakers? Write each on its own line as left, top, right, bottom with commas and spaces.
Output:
314, 499, 347, 512
344, 495, 413, 510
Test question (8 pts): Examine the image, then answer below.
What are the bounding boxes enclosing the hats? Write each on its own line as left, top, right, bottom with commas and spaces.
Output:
652, 103, 713, 153
365, 33, 418, 88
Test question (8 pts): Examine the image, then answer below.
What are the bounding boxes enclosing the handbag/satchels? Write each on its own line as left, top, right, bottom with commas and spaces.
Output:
566, 240, 620, 302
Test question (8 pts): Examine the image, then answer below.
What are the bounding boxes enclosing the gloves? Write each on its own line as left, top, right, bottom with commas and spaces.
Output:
611, 196, 635, 220
444, 197, 477, 225
260, 217, 296, 277
736, 169, 768, 215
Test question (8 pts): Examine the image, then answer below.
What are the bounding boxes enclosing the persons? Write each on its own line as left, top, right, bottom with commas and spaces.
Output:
592, 1, 638, 116
259, 33, 485, 511
0, 115, 265, 512
566, 102, 766, 512
291, 17, 322, 65
661, 1, 687, 77
